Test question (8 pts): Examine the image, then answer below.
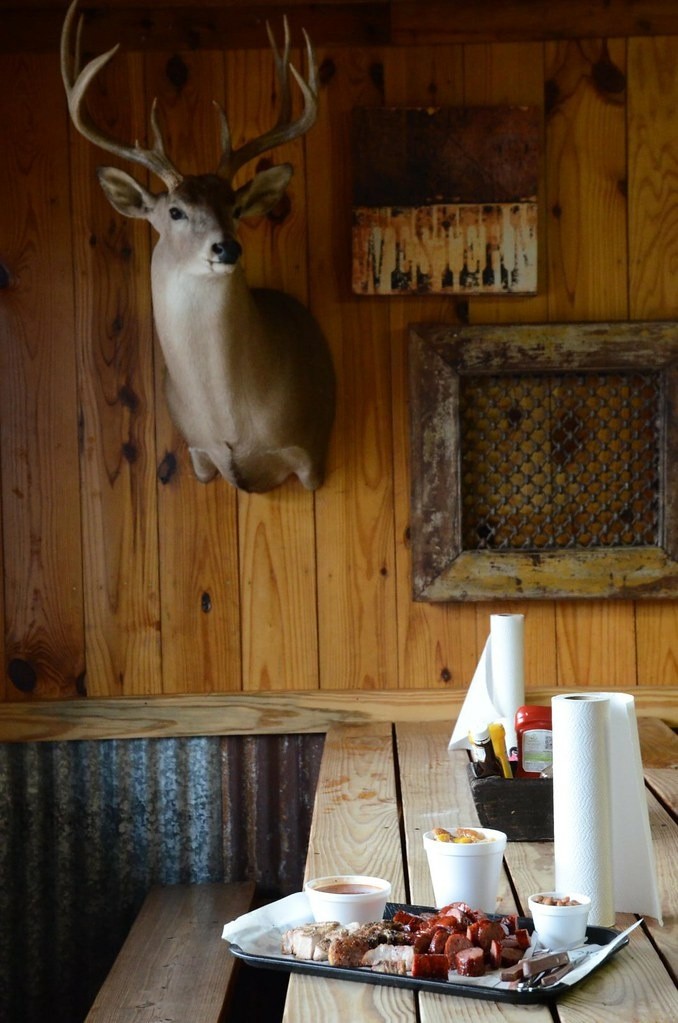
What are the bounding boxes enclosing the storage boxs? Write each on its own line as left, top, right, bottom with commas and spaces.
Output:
467, 762, 554, 842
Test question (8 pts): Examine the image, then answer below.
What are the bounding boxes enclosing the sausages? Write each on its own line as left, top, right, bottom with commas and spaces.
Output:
394, 901, 532, 982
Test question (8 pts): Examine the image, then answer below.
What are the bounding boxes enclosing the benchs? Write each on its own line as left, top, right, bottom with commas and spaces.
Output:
84, 881, 256, 1023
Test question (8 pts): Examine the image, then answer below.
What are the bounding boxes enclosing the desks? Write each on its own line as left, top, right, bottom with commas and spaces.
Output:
281, 720, 678, 1023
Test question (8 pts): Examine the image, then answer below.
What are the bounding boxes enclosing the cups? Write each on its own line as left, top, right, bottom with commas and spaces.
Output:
423, 826, 507, 919
527, 891, 593, 950
305, 876, 392, 931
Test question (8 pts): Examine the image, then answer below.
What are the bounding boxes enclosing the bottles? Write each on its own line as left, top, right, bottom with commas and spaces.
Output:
515, 704, 558, 779
468, 726, 505, 778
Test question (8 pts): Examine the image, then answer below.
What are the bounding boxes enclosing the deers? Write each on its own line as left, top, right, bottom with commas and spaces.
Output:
60, 1, 336, 495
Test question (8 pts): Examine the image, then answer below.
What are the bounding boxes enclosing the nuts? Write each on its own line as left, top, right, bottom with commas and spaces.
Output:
536, 895, 580, 907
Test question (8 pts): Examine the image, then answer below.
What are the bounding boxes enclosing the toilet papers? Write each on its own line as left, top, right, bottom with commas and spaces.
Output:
550, 692, 666, 929
446, 611, 525, 759
